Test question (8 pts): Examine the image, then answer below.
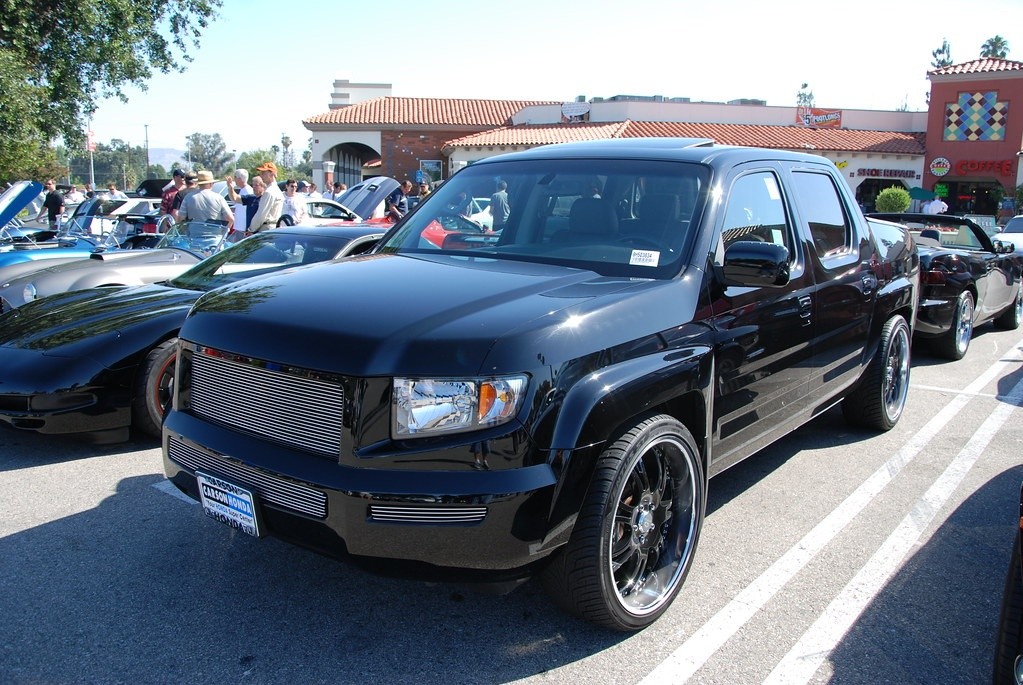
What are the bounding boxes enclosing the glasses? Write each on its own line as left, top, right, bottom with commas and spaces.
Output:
290, 185, 297, 187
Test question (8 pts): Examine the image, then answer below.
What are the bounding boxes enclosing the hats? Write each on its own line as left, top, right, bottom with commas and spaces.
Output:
419, 180, 428, 186
299, 180, 311, 188
184, 171, 198, 182
191, 170, 220, 184
256, 162, 278, 177
173, 169, 185, 177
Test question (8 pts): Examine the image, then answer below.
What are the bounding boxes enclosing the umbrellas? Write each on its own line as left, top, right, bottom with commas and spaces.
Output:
906, 187, 937, 212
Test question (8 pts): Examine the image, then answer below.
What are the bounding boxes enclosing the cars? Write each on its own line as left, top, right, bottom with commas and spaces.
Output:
161, 137, 921, 632
996, 216, 1012, 228
547, 195, 582, 217
990, 215, 1023, 256
0, 226, 444, 440
863, 213, 1023, 359
304, 176, 496, 251
0, 181, 307, 313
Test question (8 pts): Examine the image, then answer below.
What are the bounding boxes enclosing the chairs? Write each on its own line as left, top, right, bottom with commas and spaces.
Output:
549, 194, 631, 250
920, 229, 941, 243
632, 196, 691, 251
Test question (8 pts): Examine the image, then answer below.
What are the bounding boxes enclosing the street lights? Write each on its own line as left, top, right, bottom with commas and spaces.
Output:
66, 158, 71, 185
232, 149, 237, 170
144, 124, 150, 180
122, 164, 126, 191
186, 136, 192, 174
282, 132, 285, 167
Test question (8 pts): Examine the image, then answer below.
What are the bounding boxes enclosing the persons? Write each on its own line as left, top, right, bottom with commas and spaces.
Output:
458, 192, 472, 229
591, 187, 602, 198
368, 198, 386, 220
389, 180, 412, 221
244, 162, 284, 245
160, 168, 201, 233
178, 171, 235, 251
920, 195, 948, 215
27, 184, 95, 216
469, 438, 492, 470
995, 242, 1014, 253
226, 169, 348, 237
36, 179, 65, 230
106, 182, 128, 200
0, 186, 5, 196
489, 180, 512, 231
418, 181, 431, 202
